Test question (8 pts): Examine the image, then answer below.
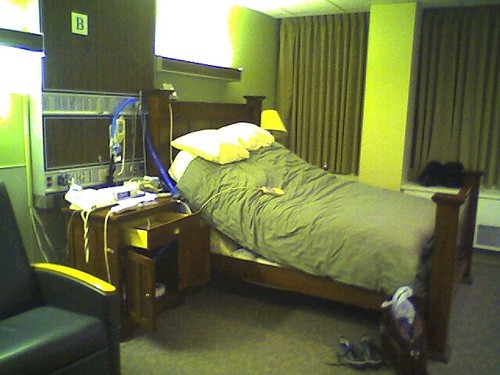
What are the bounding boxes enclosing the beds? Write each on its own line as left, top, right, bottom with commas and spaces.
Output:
144, 89, 486, 368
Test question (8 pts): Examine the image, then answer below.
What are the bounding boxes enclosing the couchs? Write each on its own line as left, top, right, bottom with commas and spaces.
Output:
0, 177, 127, 375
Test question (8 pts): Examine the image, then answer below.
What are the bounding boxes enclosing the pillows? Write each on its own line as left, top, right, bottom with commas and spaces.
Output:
170, 125, 252, 164
217, 120, 276, 153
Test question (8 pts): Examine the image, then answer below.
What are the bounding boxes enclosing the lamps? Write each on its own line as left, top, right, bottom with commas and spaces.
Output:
259, 107, 288, 144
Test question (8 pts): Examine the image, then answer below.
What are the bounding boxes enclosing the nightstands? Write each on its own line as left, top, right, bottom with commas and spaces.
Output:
63, 183, 215, 343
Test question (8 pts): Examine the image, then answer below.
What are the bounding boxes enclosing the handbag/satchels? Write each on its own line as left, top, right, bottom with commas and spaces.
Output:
380, 299, 426, 374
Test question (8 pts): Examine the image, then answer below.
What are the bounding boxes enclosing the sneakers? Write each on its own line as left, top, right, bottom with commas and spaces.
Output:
360, 335, 385, 368
327, 336, 365, 366
